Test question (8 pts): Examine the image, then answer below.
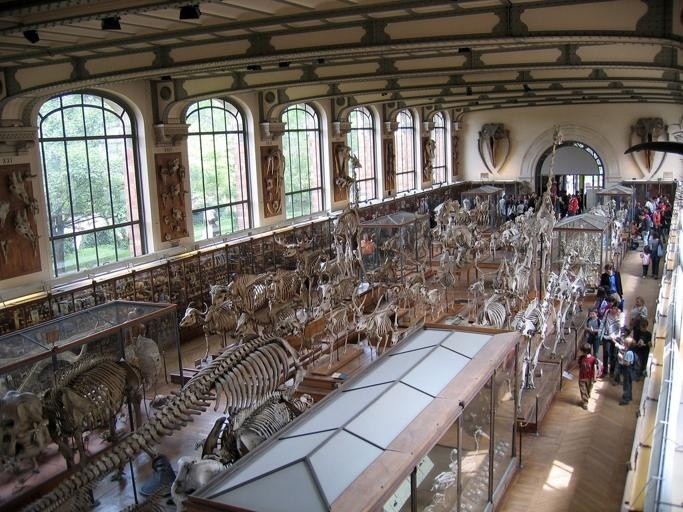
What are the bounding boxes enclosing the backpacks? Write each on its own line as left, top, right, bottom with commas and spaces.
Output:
628, 352, 641, 371
579, 356, 603, 377
598, 297, 607, 316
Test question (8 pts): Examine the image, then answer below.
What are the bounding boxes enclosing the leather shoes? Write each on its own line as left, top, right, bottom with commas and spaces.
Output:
597, 372, 632, 406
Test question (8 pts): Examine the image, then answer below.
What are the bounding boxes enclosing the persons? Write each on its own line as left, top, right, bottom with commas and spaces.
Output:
361, 233, 377, 283
634, 193, 672, 279
566, 264, 652, 407
498, 189, 586, 220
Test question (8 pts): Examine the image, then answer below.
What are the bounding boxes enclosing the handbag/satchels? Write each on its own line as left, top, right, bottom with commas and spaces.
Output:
658, 243, 664, 257
